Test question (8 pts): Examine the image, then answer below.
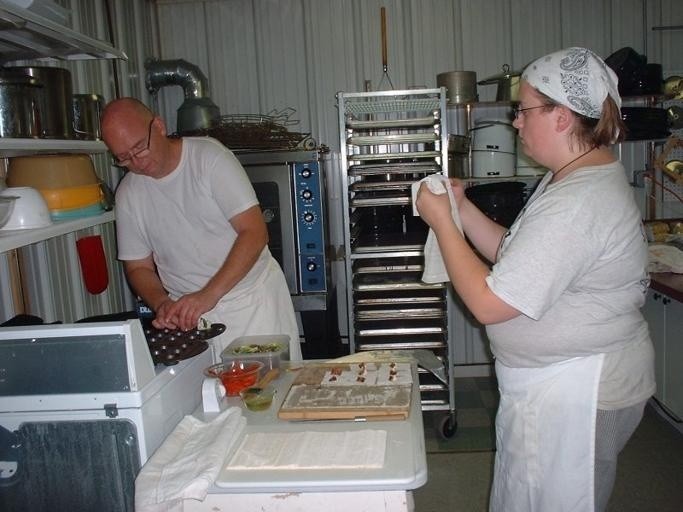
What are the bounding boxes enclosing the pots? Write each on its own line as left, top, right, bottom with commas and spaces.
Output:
620, 107, 672, 142
436, 70, 517, 179
1, 67, 105, 139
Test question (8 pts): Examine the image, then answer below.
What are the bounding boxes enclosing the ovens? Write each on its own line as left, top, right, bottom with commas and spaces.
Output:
233, 150, 331, 295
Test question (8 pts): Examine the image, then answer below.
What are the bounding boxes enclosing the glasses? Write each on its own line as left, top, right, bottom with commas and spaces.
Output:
114, 118, 154, 168
513, 105, 553, 120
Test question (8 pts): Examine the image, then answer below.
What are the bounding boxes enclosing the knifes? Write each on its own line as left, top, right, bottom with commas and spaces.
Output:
287, 415, 406, 423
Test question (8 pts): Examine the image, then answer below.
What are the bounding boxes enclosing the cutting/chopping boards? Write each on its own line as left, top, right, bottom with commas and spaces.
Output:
277, 361, 415, 420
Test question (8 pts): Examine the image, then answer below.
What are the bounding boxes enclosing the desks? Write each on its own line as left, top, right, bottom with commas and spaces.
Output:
181, 360, 429, 512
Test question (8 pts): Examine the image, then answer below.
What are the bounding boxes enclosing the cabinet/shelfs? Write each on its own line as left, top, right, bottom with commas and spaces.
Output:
446, 282, 495, 378
640, 286, 683, 426
334, 85, 459, 441
445, 100, 544, 182
2, 0, 128, 255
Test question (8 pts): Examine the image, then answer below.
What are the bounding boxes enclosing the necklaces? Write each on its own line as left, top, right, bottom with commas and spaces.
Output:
540, 146, 596, 192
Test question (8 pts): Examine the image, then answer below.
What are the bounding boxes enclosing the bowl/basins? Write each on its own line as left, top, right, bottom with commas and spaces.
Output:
665, 76, 683, 175
202, 360, 276, 412
604, 45, 664, 98
0, 155, 107, 232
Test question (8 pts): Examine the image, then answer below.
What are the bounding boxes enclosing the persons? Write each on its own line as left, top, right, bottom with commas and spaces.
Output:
99, 96, 303, 369
412, 47, 657, 512
